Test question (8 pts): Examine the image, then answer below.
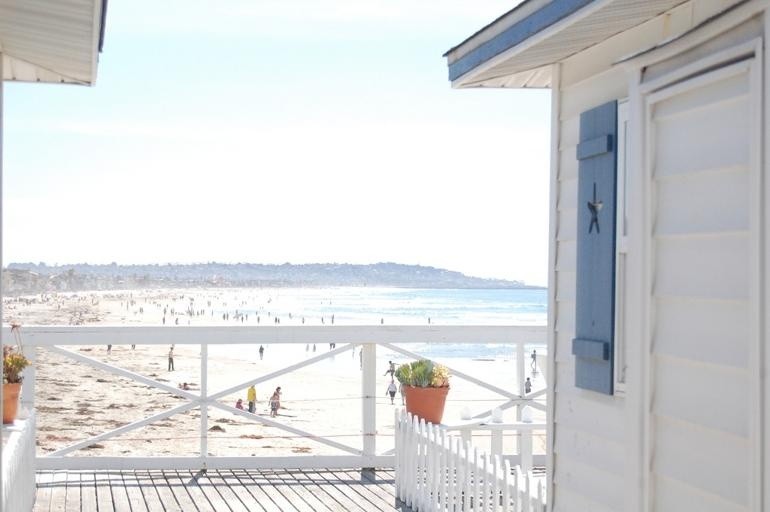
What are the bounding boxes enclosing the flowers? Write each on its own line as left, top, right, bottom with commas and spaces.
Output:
2, 347, 32, 382
393, 359, 452, 387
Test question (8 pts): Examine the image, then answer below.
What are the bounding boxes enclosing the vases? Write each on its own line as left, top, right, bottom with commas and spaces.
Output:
405, 387, 450, 424
3, 383, 22, 422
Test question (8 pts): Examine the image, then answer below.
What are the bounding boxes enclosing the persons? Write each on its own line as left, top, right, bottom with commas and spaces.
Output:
531, 349, 537, 372
235, 384, 283, 416
525, 376, 533, 394
384, 361, 401, 379
386, 379, 397, 405
2, 289, 384, 372
